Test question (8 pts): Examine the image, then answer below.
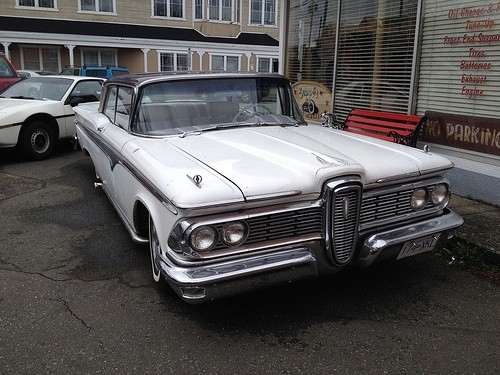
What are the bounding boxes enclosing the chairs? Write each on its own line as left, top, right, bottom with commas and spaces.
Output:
171, 101, 208, 134
139, 102, 173, 137
205, 101, 242, 129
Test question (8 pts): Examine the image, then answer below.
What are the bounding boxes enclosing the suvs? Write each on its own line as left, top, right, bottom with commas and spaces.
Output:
62, 64, 131, 79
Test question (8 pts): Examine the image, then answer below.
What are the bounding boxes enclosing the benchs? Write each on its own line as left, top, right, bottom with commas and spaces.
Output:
321, 107, 428, 148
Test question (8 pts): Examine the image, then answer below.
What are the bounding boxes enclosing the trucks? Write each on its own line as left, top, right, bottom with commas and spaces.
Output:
0, 52, 21, 93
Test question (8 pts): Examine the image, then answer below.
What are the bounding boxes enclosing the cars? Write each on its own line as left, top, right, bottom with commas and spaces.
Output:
72, 72, 463, 300
16, 69, 60, 78
0, 75, 153, 161
